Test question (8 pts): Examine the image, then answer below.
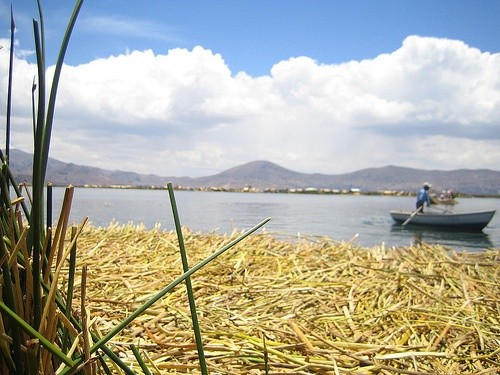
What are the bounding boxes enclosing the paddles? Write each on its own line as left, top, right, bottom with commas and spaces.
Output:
431, 206, 456, 214
402, 205, 424, 226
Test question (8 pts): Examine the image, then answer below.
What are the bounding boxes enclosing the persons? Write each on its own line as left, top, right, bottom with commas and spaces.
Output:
416, 182, 432, 213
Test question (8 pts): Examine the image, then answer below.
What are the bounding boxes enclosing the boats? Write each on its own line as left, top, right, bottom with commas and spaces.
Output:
428, 194, 455, 205
389, 209, 496, 229
